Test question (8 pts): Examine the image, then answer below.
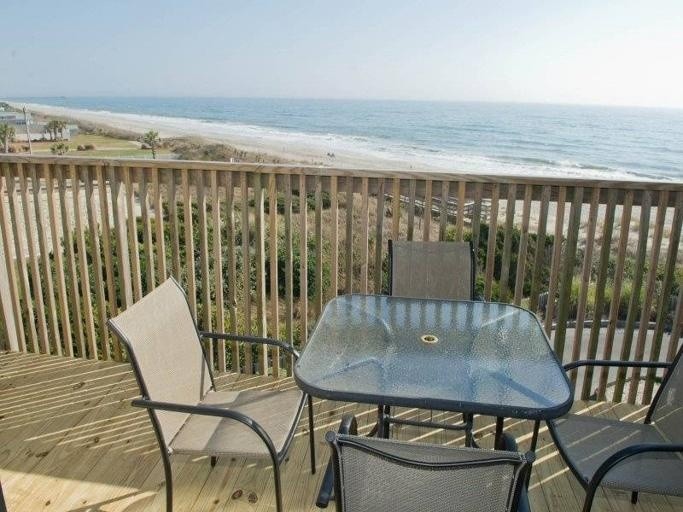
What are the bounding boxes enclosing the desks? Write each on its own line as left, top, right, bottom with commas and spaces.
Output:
294, 294, 573, 491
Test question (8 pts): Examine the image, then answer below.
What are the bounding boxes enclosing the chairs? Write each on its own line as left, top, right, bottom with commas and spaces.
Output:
315, 413, 536, 512
106, 276, 316, 512
388, 240, 475, 428
532, 345, 683, 511
